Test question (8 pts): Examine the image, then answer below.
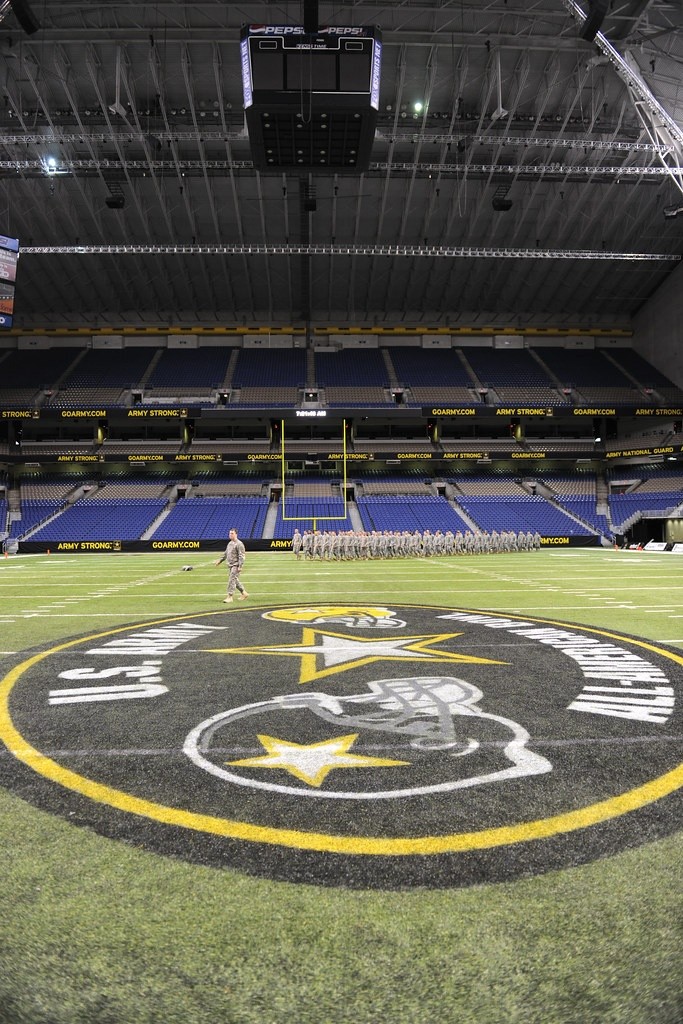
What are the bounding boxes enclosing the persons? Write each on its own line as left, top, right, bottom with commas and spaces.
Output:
215, 528, 249, 603
302, 530, 541, 562
291, 529, 302, 560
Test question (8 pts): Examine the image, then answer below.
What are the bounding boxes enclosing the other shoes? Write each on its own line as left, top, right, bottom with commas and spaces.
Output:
223, 595, 234, 604
238, 591, 249, 601
